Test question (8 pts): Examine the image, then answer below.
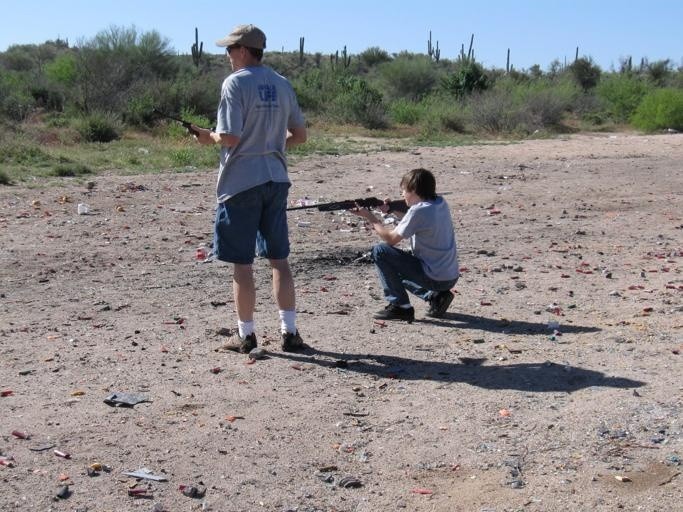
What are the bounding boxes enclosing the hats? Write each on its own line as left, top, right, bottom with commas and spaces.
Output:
215, 24, 267, 49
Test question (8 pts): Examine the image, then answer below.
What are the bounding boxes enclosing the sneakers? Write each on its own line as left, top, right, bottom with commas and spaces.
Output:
221, 329, 257, 354
281, 328, 303, 352
372, 303, 414, 321
423, 289, 454, 316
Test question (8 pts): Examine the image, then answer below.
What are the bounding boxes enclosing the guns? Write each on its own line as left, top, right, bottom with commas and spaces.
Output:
286, 196, 408, 213
150, 106, 216, 135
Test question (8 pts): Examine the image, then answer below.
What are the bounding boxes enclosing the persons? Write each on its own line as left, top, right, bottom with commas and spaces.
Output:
349, 167, 459, 323
188, 23, 311, 354
